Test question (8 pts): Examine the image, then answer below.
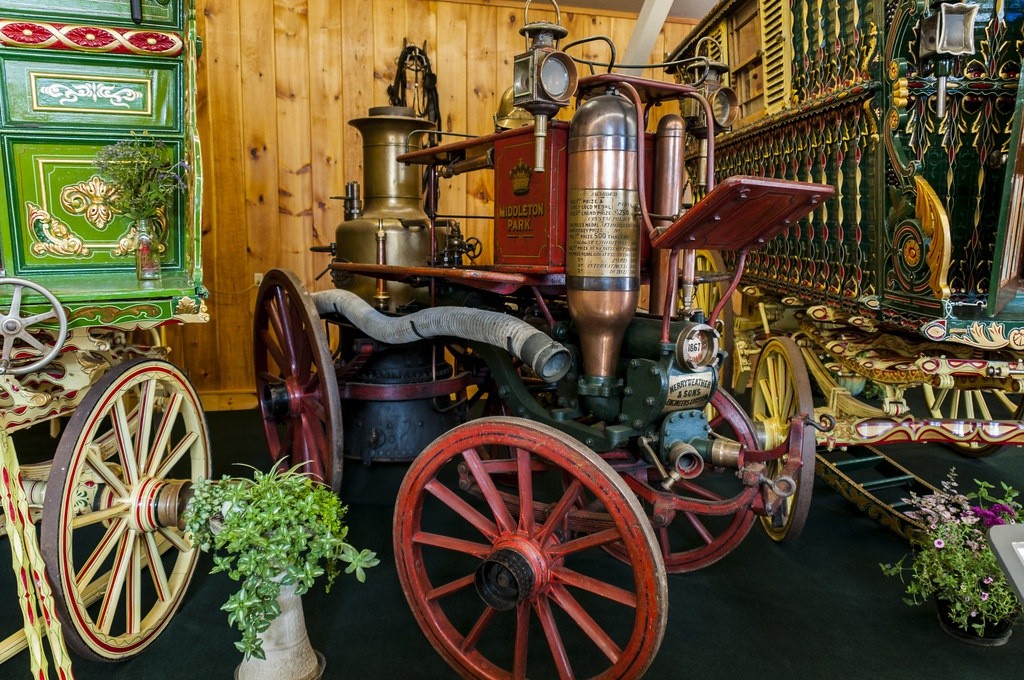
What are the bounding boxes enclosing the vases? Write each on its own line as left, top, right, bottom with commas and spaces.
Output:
136, 220, 161, 281
934, 580, 1018, 639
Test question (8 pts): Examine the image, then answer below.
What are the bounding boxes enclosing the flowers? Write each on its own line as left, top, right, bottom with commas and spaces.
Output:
89, 131, 194, 276
879, 466, 1024, 637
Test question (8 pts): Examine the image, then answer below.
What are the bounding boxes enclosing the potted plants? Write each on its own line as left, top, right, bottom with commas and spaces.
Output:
184, 453, 381, 680
